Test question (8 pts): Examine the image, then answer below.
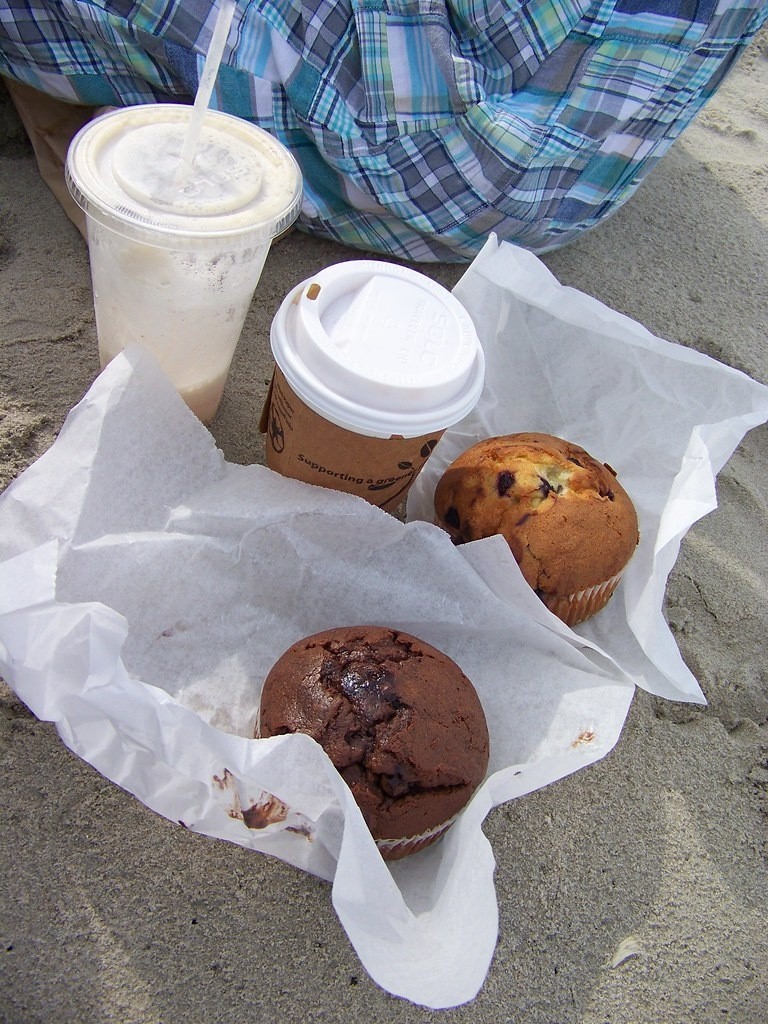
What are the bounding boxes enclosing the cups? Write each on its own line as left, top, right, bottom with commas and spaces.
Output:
250, 257, 488, 514
64, 103, 305, 431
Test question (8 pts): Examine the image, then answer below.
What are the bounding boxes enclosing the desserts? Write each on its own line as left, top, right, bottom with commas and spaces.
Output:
433, 433, 642, 626
256, 626, 490, 862
